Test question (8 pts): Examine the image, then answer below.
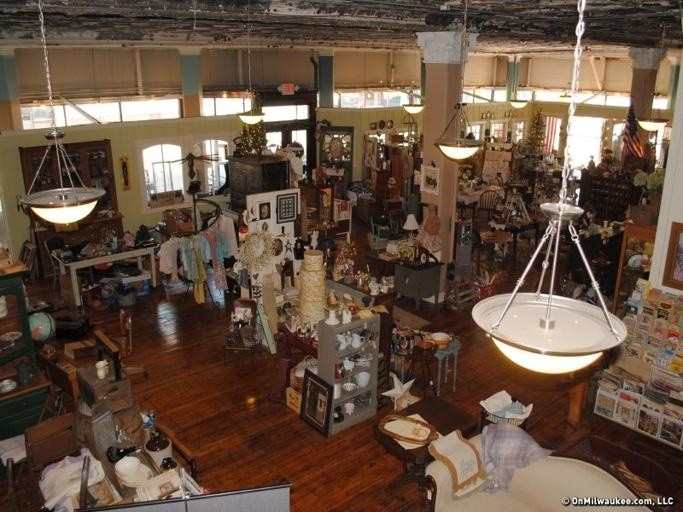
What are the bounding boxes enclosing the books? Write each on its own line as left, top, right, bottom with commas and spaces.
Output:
594, 279, 683, 451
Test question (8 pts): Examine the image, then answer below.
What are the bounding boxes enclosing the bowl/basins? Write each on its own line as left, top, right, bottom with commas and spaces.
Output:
343, 383, 355, 392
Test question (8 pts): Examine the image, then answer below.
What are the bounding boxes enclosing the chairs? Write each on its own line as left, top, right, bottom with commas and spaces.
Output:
477, 190, 499, 220
224, 301, 258, 365
42, 236, 92, 290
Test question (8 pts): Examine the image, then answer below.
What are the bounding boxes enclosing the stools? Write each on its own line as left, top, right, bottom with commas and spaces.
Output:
400, 351, 435, 400
431, 338, 461, 398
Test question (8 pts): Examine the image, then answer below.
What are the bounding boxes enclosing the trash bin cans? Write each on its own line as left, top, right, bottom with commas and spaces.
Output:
481, 398, 527, 432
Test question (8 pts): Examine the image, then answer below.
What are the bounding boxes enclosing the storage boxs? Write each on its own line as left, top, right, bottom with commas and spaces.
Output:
77, 366, 134, 414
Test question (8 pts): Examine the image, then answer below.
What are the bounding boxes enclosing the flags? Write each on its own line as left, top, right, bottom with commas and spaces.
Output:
622, 103, 644, 158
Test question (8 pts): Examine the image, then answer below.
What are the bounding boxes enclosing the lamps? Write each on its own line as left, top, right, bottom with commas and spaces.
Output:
404, 34, 425, 115
509, 25, 528, 109
471, 0, 627, 376
17, 1, 107, 226
434, 0, 485, 163
236, 0, 265, 125
402, 214, 420, 238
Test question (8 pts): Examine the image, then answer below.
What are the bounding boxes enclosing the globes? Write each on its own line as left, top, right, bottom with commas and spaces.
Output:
28, 312, 58, 357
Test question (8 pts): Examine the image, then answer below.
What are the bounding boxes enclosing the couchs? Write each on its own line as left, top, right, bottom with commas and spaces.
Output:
424, 424, 661, 512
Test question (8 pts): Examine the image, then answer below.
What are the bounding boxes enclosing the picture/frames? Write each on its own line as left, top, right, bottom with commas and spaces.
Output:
419, 164, 440, 195
661, 221, 682, 290
298, 367, 334, 438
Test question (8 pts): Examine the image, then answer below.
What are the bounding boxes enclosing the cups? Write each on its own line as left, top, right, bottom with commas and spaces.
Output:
354, 372, 370, 388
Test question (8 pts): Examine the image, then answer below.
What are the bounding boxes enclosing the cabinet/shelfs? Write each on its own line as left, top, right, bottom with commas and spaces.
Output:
225, 155, 290, 215
318, 311, 381, 436
0, 274, 55, 442
19, 139, 126, 280
354, 137, 404, 227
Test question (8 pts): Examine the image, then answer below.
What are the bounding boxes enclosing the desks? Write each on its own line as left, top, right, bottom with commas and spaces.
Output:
373, 397, 478, 480
62, 239, 159, 309
503, 222, 542, 247
